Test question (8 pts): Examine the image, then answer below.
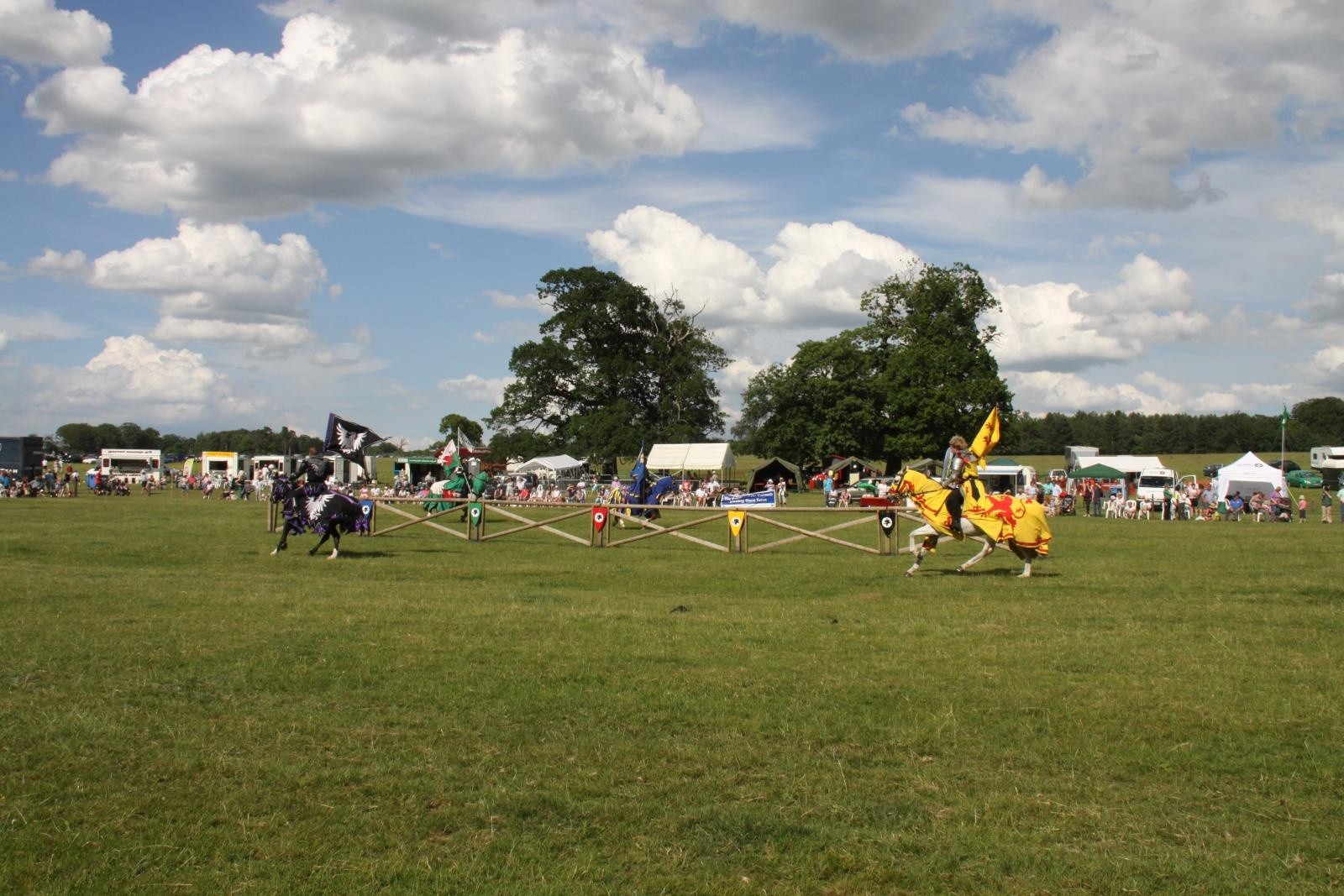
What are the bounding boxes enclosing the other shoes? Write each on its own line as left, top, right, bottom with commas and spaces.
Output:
948, 524, 961, 533
1083, 513, 1091, 517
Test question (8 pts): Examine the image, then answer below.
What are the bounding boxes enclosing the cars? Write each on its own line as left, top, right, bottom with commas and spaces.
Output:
1047, 469, 1068, 481
1268, 459, 1302, 472
1284, 470, 1324, 490
60, 450, 83, 464
827, 475, 895, 507
1204, 463, 1228, 478
493, 472, 538, 494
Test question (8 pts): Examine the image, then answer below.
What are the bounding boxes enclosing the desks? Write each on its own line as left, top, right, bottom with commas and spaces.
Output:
215, 478, 227, 488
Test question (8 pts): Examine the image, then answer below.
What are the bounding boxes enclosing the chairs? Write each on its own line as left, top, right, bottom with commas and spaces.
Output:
353, 484, 1205, 520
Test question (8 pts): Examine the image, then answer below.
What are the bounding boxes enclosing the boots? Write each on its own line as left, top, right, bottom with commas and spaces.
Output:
285, 497, 299, 517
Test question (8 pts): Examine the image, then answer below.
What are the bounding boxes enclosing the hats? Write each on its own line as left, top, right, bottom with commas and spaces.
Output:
613, 477, 619, 481
780, 477, 783, 480
713, 471, 715, 473
1299, 495, 1304, 499
768, 479, 773, 483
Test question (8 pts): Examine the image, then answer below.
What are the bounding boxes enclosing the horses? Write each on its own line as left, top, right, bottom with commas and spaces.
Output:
270, 472, 365, 560
427, 471, 494, 522
608, 474, 680, 530
886, 466, 1042, 578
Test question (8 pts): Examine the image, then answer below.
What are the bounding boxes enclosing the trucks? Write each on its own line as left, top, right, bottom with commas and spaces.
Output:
1064, 445, 1099, 477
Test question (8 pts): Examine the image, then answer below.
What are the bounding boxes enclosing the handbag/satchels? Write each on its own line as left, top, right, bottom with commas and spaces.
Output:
785, 493, 789, 497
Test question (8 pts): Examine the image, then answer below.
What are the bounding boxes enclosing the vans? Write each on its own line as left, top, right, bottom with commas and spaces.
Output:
1136, 468, 1199, 513
85, 448, 164, 484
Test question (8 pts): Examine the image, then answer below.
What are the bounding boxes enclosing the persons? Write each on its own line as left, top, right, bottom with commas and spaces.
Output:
0, 459, 355, 531
940, 435, 974, 539
359, 468, 1344, 524
626, 457, 657, 496
283, 447, 329, 516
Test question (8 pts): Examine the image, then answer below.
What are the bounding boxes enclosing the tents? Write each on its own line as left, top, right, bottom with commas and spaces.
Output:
513, 442, 1283, 508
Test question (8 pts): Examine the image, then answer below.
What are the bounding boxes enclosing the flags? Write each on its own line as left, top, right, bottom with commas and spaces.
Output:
323, 412, 392, 471
438, 438, 462, 478
972, 408, 1000, 470
631, 442, 645, 485
459, 429, 475, 453
